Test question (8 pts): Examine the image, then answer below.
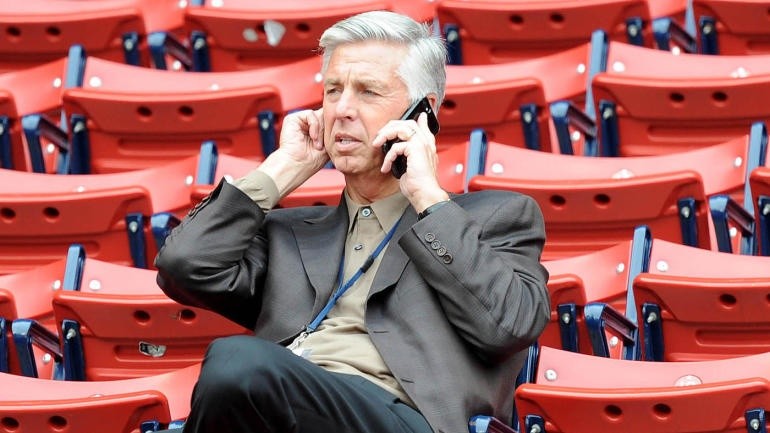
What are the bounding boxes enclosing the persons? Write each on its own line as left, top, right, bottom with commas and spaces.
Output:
152, 11, 550, 433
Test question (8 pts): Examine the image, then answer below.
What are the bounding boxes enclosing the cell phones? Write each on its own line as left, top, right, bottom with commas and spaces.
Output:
381, 97, 441, 179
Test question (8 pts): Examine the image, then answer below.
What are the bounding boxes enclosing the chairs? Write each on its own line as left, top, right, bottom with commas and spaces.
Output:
0, 0, 770, 432
